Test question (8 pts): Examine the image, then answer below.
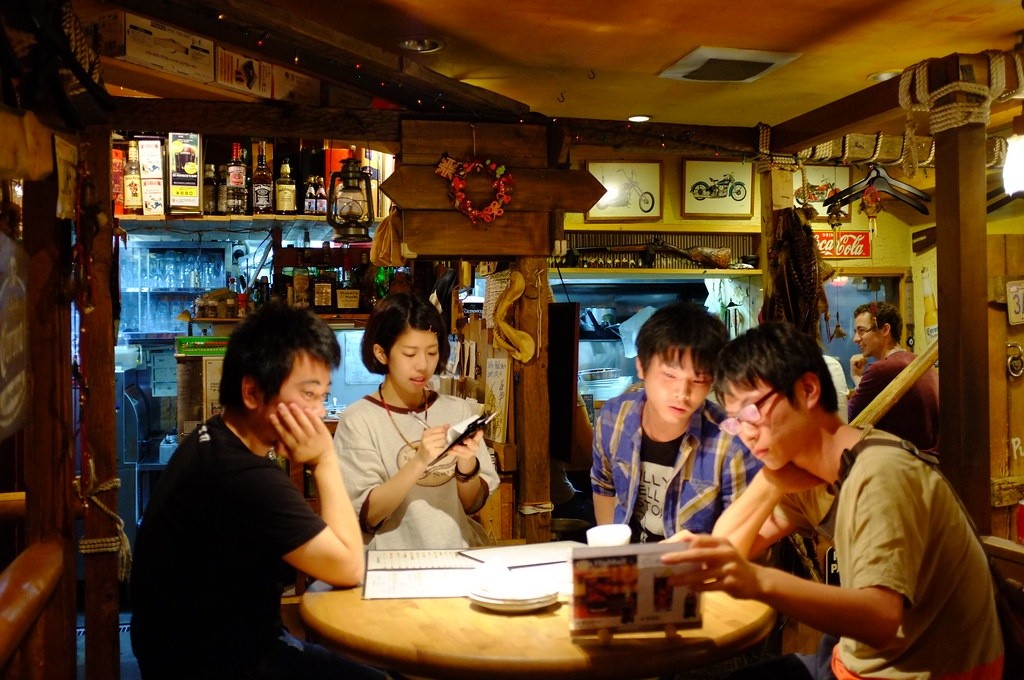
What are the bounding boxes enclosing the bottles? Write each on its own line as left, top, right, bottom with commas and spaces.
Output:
198, 276, 269, 317
203, 139, 327, 216
286, 241, 393, 314
620, 579, 638, 625
683, 587, 696, 619
122, 140, 143, 214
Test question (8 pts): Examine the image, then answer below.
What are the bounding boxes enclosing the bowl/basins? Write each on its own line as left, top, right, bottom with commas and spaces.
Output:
577, 367, 634, 401
325, 405, 347, 420
114, 344, 140, 370
585, 307, 616, 326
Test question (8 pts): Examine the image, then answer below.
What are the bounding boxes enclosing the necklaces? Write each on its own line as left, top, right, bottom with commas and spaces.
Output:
379, 382, 427, 451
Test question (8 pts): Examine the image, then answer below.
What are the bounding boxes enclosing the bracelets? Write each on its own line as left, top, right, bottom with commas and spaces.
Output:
455, 457, 480, 483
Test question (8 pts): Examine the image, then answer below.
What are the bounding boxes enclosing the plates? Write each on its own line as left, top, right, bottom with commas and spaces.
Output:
469, 574, 558, 614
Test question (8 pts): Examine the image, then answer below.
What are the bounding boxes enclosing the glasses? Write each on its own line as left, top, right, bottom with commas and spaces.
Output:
854, 326, 875, 336
717, 387, 778, 437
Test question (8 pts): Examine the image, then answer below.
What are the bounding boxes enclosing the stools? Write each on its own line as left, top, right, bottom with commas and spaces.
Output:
550, 518, 592, 540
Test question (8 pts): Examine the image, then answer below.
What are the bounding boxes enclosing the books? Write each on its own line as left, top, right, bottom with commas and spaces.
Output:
361, 541, 566, 599
570, 540, 703, 637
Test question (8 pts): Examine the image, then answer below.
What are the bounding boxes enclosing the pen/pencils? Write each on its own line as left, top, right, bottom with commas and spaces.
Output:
411, 411, 432, 429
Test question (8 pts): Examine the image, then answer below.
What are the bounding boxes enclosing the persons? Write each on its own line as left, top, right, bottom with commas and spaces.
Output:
589, 302, 766, 542
334, 292, 500, 551
850, 302, 938, 448
660, 321, 1004, 680
551, 394, 595, 518
822, 355, 849, 424
130, 303, 386, 680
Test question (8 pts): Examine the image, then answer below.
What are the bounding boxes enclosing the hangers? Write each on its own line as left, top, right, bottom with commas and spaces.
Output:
822, 166, 932, 216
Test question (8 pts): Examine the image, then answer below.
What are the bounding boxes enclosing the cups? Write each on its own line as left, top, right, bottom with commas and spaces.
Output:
584, 573, 610, 612
587, 523, 633, 548
653, 566, 674, 613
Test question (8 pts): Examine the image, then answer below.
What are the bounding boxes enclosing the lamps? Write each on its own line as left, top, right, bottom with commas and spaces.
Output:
1003, 114, 1024, 198
326, 159, 375, 243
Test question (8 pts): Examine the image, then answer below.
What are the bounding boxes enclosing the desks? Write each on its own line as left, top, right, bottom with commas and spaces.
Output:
290, 418, 340, 595
299, 574, 777, 680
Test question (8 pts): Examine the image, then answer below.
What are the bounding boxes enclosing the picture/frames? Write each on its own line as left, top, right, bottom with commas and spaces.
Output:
584, 159, 663, 222
794, 163, 853, 224
681, 155, 754, 220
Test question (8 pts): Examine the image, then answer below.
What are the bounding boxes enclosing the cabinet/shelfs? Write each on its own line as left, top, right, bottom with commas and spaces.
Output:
118, 240, 232, 340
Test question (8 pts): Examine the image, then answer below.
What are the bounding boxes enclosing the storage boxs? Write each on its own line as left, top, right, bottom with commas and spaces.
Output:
159, 434, 178, 462
112, 132, 203, 217
82, 9, 375, 110
